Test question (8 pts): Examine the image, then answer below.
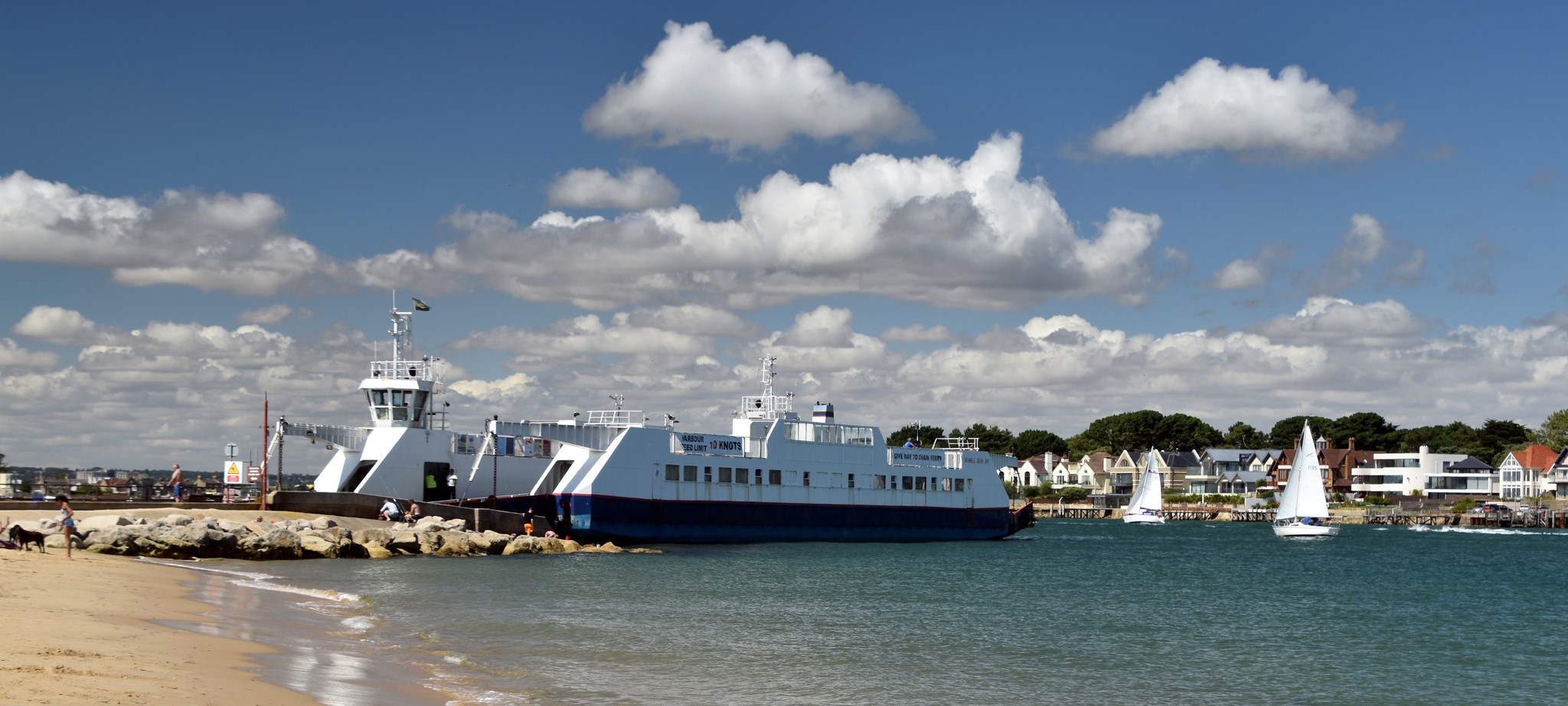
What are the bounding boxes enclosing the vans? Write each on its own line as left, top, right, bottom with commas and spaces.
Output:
1471, 504, 1496, 513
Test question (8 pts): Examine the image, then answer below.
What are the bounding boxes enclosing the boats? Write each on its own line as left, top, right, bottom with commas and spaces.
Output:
252, 287, 566, 508
456, 354, 1035, 546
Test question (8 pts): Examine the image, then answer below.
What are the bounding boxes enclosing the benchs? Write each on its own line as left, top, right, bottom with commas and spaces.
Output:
187, 494, 224, 502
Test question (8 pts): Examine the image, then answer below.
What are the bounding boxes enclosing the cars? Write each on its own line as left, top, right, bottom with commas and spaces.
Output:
1484, 504, 1514, 514
1536, 505, 1549, 512
1519, 504, 1531, 512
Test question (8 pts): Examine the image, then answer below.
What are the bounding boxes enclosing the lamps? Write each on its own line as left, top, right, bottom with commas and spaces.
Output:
327, 445, 333, 450
666, 413, 676, 420
573, 413, 580, 417
673, 421, 679, 423
307, 431, 313, 435
445, 401, 450, 407
642, 418, 650, 421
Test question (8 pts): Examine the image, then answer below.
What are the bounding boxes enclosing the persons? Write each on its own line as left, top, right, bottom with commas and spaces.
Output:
445, 469, 458, 500
970, 444, 977, 451
379, 499, 399, 521
55, 496, 77, 559
251, 517, 263, 522
424, 470, 437, 502
522, 507, 535, 535
405, 499, 421, 523
167, 463, 183, 503
903, 438, 922, 449
545, 530, 559, 538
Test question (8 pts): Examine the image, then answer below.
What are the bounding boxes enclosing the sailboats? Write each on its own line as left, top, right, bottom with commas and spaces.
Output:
1272, 418, 1342, 537
1123, 446, 1166, 524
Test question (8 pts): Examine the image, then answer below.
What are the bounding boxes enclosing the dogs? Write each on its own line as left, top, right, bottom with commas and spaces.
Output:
9, 525, 21, 544
15, 527, 45, 553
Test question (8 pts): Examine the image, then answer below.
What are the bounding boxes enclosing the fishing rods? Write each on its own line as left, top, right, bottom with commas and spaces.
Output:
374, 474, 412, 525
